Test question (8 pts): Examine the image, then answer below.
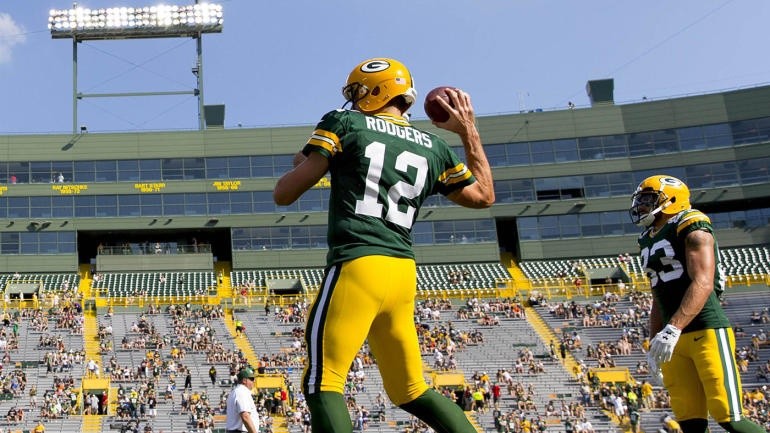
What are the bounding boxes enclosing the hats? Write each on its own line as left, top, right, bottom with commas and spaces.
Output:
237, 367, 259, 378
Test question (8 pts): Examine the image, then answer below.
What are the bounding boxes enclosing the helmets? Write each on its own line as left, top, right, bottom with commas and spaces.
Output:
342, 58, 418, 116
628, 174, 692, 226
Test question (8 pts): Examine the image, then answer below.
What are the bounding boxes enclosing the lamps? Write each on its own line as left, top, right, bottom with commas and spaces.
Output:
47, 0, 224, 39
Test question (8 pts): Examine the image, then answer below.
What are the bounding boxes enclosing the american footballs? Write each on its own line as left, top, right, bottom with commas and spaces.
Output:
423, 86, 461, 124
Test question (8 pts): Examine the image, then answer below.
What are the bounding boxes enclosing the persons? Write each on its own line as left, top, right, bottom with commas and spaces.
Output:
1, 233, 770, 433
626, 174, 768, 432
272, 57, 495, 433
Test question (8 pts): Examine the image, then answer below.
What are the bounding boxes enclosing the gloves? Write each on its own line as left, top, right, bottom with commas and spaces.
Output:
647, 352, 663, 386
649, 323, 682, 364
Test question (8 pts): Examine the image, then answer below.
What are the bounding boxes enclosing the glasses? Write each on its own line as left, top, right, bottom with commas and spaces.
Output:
247, 377, 254, 381
